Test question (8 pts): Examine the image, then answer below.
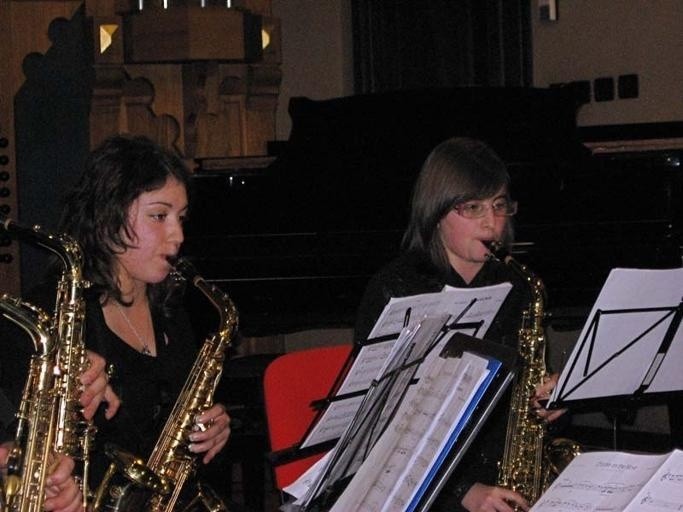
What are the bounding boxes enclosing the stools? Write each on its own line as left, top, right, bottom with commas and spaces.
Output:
207, 354, 284, 512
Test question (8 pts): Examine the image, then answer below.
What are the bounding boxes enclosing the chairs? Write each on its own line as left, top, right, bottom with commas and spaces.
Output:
265, 343, 353, 512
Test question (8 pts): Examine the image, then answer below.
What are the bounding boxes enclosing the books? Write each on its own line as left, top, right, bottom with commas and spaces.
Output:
546, 263, 683, 410
527, 442, 683, 512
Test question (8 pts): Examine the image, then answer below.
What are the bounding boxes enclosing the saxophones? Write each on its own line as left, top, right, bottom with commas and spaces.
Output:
483, 237, 581, 510
0, 216, 164, 512
88, 255, 242, 512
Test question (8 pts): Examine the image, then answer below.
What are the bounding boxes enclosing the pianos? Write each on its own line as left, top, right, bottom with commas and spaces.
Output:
163, 80, 683, 336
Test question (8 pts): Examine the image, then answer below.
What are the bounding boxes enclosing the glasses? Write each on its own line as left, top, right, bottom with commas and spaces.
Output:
453, 199, 518, 219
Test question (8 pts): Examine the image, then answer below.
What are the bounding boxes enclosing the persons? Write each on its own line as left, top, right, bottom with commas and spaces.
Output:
0, 324, 122, 512
350, 136, 571, 512
0, 131, 251, 512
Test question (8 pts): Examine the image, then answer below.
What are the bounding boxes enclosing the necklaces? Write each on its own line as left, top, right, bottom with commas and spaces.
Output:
111, 295, 154, 356
